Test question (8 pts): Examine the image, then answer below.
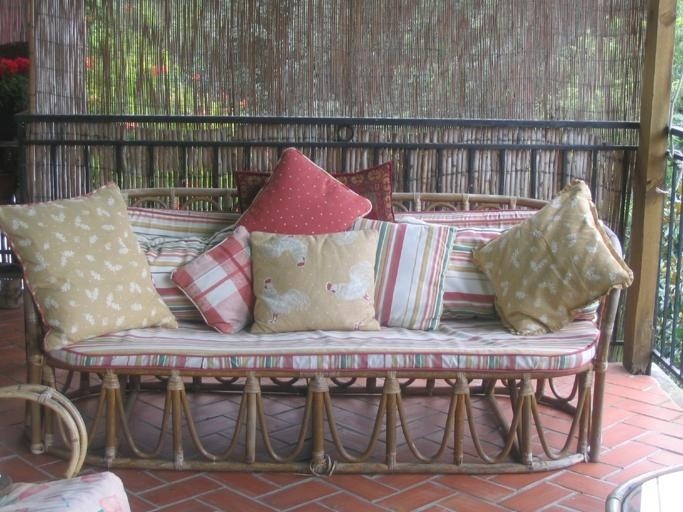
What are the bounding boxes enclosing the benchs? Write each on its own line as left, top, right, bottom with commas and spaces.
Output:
24, 186, 623, 473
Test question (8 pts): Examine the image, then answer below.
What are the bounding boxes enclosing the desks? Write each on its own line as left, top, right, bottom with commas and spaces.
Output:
604, 463, 683, 512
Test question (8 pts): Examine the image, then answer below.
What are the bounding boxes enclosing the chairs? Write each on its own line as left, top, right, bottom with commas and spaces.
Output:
0, 383, 131, 512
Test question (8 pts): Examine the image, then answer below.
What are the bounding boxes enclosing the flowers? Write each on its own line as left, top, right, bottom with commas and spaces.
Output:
0, 56, 30, 109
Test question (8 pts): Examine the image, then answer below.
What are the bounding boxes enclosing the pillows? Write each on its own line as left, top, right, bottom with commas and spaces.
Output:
233, 160, 397, 222
347, 217, 458, 332
171, 225, 257, 336
249, 228, 382, 334
134, 229, 230, 323
233, 147, 373, 235
469, 178, 634, 339
1, 182, 179, 354
404, 217, 601, 323
